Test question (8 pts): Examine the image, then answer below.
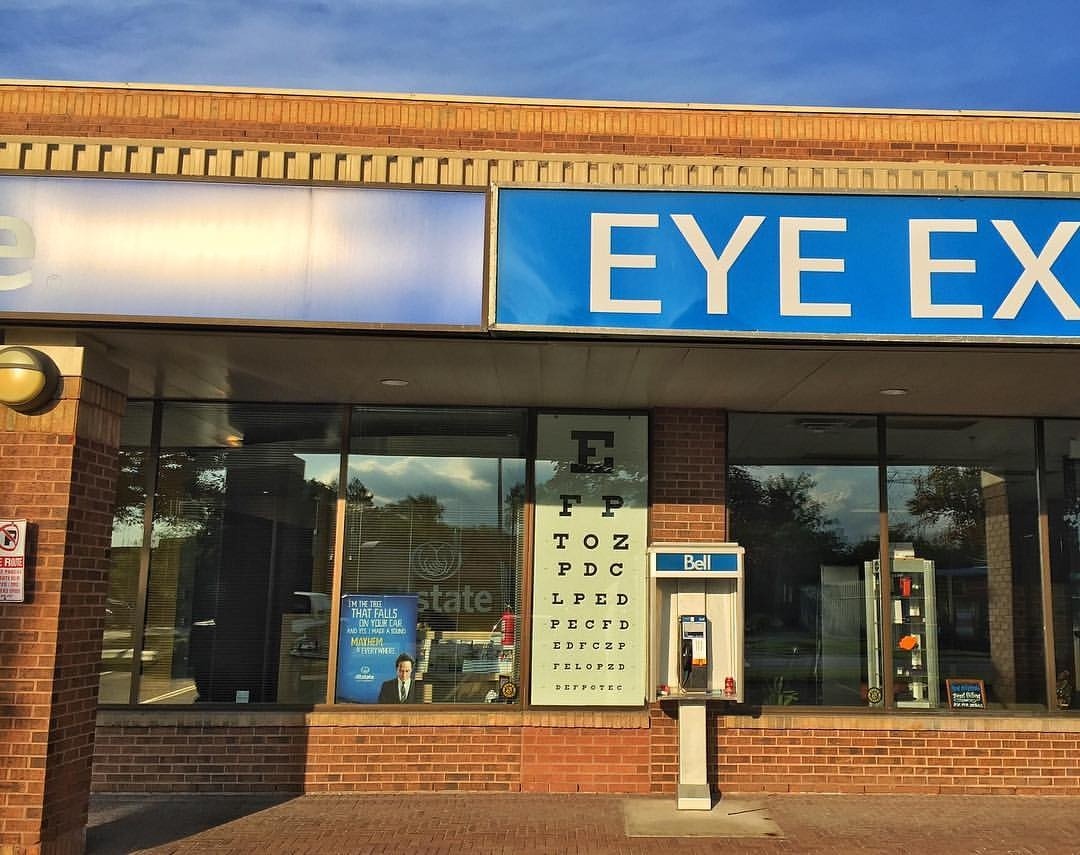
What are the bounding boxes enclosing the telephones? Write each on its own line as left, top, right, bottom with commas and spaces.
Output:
679, 614, 708, 672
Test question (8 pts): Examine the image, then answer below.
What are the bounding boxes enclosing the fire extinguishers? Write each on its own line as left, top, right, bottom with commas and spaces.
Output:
501, 601, 514, 645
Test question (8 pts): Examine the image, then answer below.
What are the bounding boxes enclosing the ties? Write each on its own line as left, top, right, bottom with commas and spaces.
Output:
401, 680, 408, 704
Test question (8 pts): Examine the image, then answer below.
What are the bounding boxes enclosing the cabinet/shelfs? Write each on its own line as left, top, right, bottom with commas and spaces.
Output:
872, 558, 927, 708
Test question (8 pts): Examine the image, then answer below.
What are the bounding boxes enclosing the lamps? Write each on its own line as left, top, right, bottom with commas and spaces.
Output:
0, 345, 59, 414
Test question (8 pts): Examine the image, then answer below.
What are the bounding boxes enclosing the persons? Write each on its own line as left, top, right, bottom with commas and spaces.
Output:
379, 654, 415, 704
